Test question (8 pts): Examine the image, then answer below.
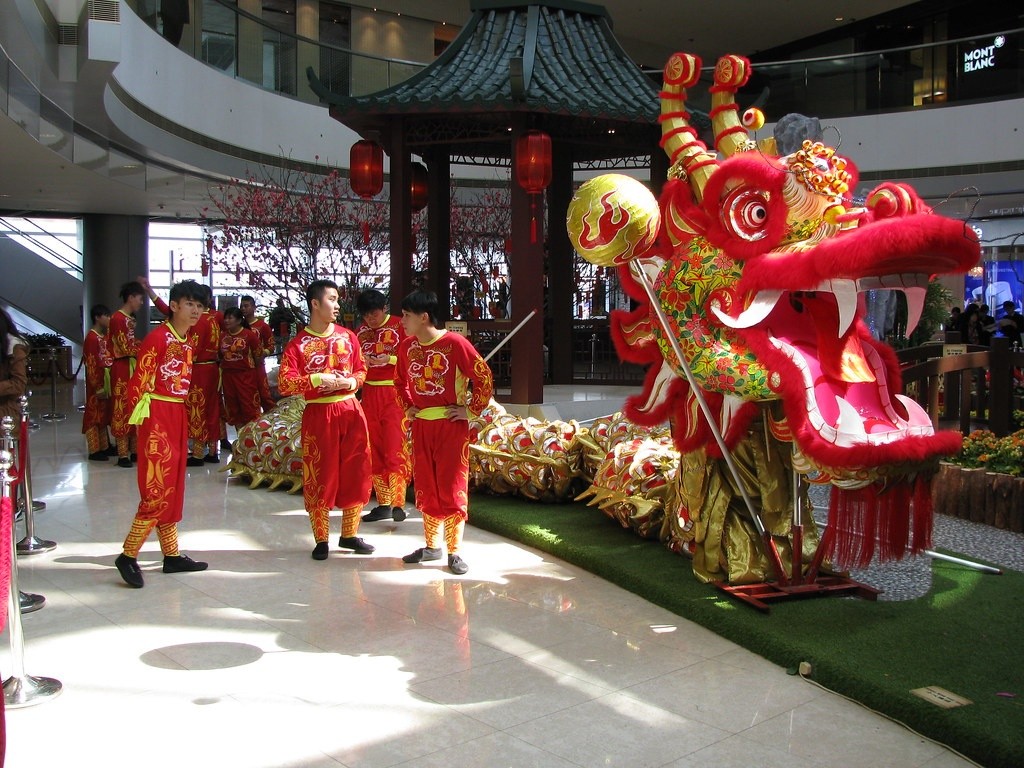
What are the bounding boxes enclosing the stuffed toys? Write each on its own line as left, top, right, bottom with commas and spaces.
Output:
609, 52, 980, 578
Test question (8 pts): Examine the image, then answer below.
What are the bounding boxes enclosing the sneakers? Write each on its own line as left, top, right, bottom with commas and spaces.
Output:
115, 553, 144, 587
163, 554, 208, 573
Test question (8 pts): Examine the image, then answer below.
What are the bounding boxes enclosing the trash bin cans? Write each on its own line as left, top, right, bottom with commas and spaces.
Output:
30, 347, 51, 377
53, 346, 72, 377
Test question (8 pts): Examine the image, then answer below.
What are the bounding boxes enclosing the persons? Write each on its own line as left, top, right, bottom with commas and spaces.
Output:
82, 282, 144, 466
269, 299, 295, 364
137, 277, 274, 466
114, 280, 209, 586
394, 289, 493, 573
0, 308, 29, 522
161, 0, 190, 45
277, 280, 375, 560
945, 301, 1024, 374
355, 288, 412, 521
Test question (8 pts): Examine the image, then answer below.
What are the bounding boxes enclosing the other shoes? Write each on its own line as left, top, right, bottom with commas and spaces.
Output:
202, 453, 220, 464
89, 451, 109, 461
103, 442, 117, 456
220, 439, 231, 449
402, 547, 442, 563
447, 555, 468, 574
15, 508, 23, 522
338, 536, 375, 552
186, 457, 204, 467
312, 542, 329, 560
392, 507, 406, 521
277, 359, 281, 365
118, 457, 133, 467
130, 454, 137, 461
362, 505, 393, 522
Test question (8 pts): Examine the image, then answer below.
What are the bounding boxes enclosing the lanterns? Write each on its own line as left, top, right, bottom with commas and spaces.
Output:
350, 139, 383, 244
411, 162, 429, 251
516, 130, 551, 245
